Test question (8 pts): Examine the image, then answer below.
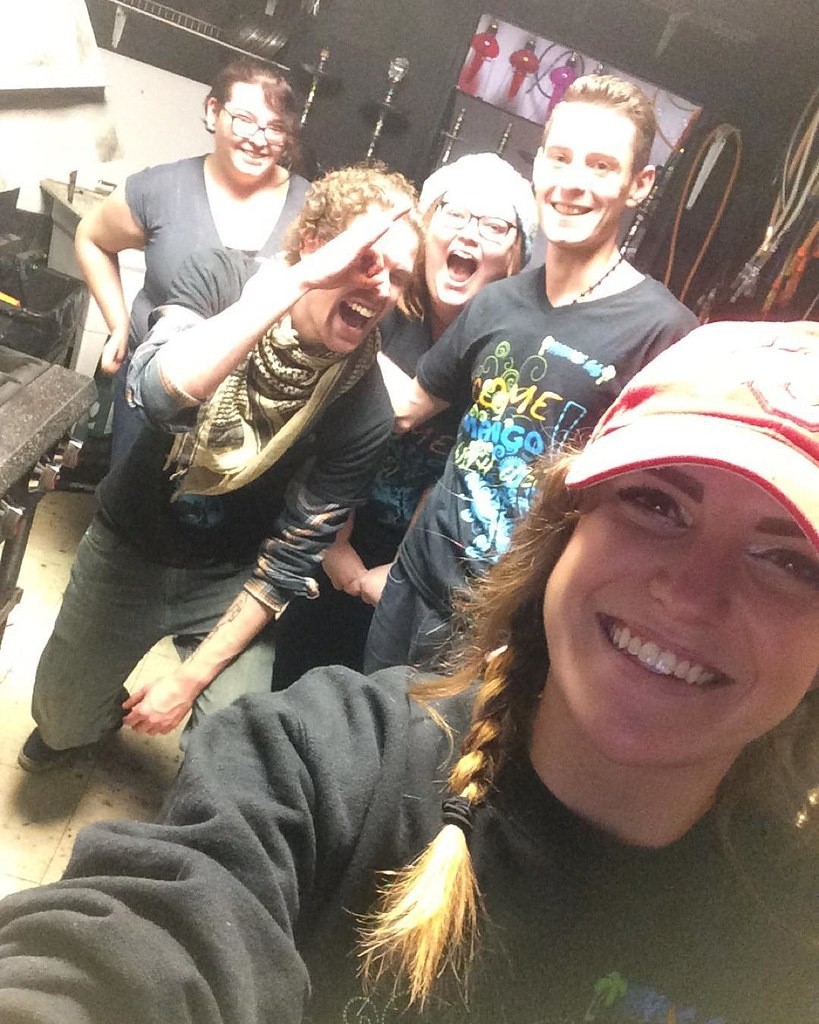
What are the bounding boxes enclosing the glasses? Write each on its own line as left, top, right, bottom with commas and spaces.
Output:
219, 97, 291, 148
434, 206, 522, 245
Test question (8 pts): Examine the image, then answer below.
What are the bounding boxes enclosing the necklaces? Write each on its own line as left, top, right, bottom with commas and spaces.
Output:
571, 255, 624, 303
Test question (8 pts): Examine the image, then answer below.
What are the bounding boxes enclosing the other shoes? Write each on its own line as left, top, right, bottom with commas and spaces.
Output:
18, 725, 80, 774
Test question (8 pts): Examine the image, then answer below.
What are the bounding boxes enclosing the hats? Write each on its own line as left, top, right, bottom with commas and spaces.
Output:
565, 320, 818, 562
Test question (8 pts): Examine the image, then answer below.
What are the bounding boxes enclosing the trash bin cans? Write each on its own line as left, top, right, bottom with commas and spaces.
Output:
0, 253, 89, 367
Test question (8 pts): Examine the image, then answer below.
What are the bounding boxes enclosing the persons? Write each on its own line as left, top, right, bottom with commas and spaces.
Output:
18, 167, 427, 771
364, 75, 698, 676
270, 152, 539, 692
75, 65, 312, 472
0, 320, 819, 1024
